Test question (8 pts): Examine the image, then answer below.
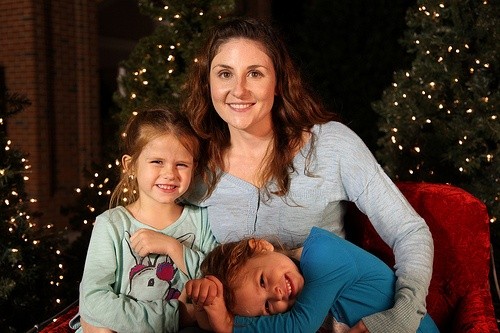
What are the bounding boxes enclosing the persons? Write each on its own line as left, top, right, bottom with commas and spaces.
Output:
73, 101, 222, 333
181, 16, 436, 333
186, 226, 439, 333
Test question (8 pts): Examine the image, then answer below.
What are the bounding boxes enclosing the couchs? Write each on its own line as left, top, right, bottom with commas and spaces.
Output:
23, 183, 500, 333
